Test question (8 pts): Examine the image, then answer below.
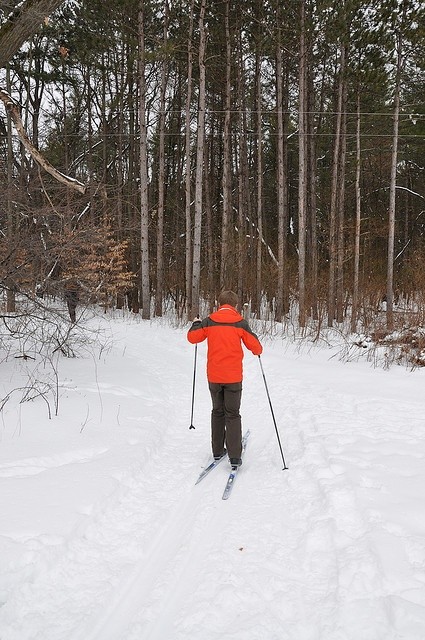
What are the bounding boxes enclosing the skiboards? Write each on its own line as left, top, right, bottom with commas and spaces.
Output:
194, 430, 251, 500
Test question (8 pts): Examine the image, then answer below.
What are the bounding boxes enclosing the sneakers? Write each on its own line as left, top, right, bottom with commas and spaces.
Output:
213, 448, 226, 460
227, 457, 242, 466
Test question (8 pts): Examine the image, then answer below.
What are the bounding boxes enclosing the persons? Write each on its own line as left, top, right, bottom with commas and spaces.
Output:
187, 290, 263, 466
66, 271, 91, 323
